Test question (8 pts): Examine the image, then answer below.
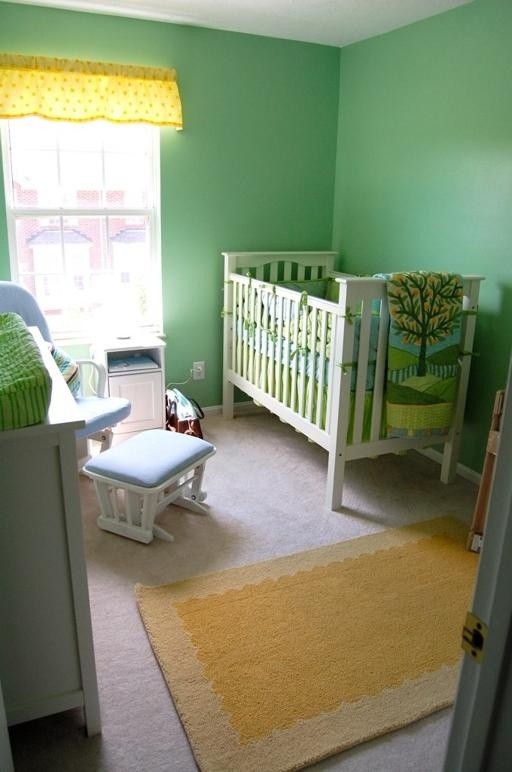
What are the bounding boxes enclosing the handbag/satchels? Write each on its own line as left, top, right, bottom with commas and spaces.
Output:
166, 395, 205, 439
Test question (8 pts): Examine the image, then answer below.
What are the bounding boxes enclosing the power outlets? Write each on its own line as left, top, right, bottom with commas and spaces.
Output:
193, 361, 205, 380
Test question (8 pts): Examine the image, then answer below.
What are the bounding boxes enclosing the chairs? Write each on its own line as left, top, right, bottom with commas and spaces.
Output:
0, 280, 131, 480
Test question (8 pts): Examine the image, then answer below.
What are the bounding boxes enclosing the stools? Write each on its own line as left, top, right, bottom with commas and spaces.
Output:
81, 428, 217, 544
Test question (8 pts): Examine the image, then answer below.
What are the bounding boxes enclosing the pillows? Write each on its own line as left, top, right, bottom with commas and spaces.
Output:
256, 284, 313, 325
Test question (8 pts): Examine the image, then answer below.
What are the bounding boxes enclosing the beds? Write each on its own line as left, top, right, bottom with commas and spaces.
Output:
221, 251, 486, 511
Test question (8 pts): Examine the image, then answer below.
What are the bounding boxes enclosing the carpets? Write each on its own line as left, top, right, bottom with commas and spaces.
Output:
133, 507, 481, 772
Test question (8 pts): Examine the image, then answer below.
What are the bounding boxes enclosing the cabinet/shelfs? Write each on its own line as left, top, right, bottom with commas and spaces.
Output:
92, 332, 167, 444
0, 326, 102, 739
